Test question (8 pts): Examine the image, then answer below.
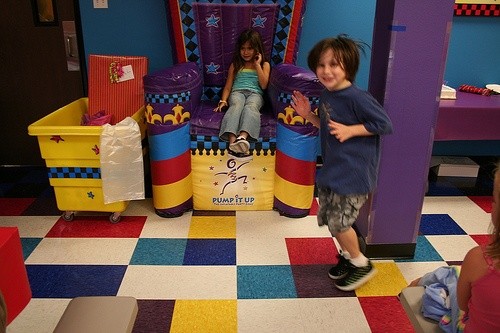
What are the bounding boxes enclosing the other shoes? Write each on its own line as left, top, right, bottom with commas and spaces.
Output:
229, 138, 250, 156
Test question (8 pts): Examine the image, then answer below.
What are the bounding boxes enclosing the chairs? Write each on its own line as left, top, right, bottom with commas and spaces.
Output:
142, 0, 322, 217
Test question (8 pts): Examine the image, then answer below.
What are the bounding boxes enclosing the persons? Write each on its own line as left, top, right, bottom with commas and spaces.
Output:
397, 171, 500, 333
213, 30, 270, 157
291, 37, 394, 290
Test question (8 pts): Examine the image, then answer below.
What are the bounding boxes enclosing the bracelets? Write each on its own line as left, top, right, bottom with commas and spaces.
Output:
218, 100, 227, 107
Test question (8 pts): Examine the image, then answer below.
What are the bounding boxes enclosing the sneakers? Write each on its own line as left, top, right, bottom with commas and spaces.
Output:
329, 255, 352, 278
335, 259, 376, 291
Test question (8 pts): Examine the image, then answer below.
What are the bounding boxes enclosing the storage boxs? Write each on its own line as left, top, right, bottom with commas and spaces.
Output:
28, 96, 147, 223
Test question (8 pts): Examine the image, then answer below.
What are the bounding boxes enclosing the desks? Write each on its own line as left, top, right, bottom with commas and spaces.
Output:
433, 88, 500, 141
